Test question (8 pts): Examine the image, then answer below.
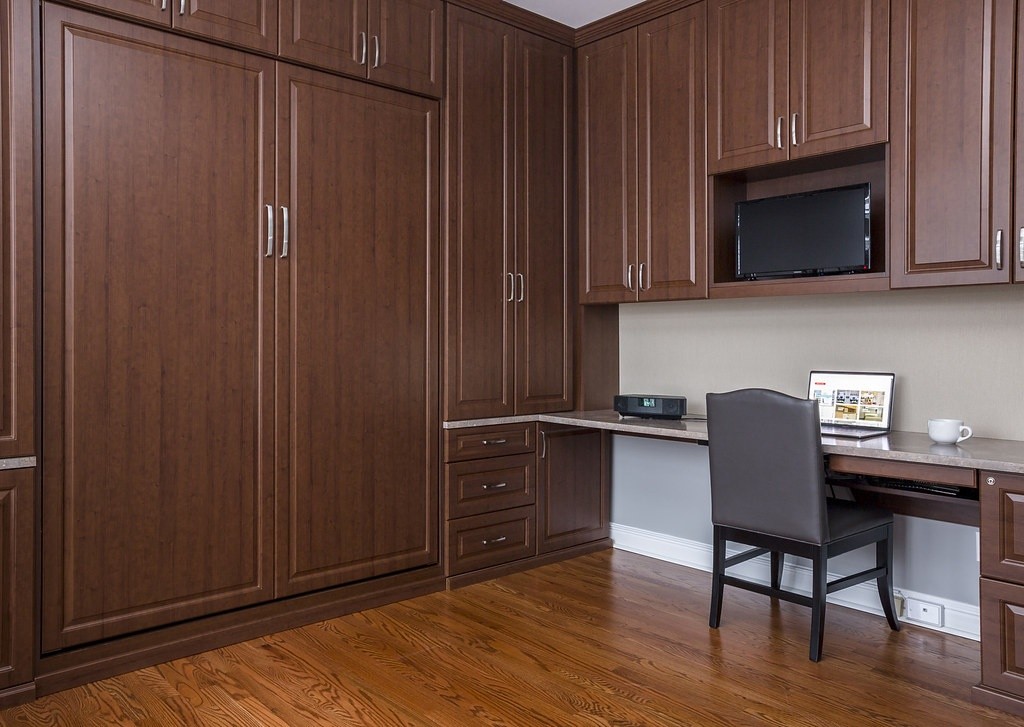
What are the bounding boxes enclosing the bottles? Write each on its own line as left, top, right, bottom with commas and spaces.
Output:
929, 418, 974, 445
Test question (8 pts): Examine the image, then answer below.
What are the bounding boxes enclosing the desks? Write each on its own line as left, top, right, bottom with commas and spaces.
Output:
441, 407, 1024, 719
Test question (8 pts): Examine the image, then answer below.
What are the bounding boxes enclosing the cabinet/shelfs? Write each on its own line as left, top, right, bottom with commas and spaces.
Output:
579, 1, 710, 302
0, 0, 621, 709
707, 0, 889, 175
889, 0, 1024, 290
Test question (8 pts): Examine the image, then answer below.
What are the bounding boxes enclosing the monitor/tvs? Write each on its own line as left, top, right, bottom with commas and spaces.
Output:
734, 182, 872, 281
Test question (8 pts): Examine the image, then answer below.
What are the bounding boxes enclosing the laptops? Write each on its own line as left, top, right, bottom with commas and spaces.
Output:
808, 371, 896, 438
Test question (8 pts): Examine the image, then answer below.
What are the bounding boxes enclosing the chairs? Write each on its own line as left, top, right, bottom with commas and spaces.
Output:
705, 388, 900, 663
